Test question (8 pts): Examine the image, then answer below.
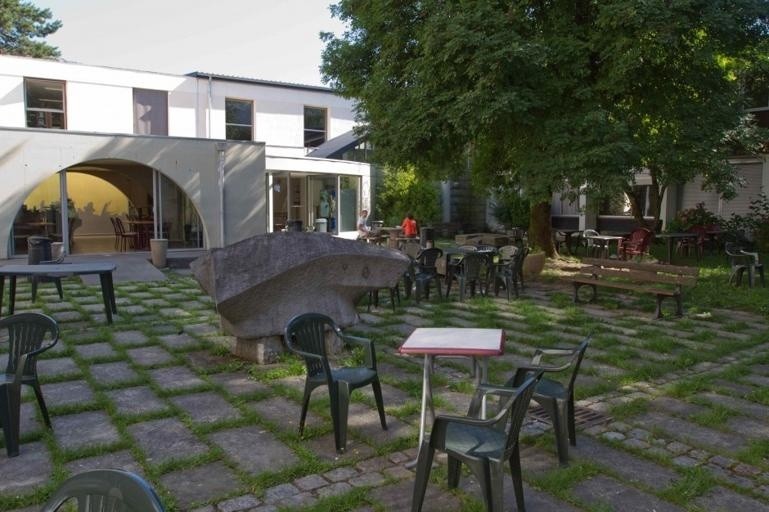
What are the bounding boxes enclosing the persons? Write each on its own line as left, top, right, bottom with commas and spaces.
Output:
319, 195, 329, 218
328, 194, 337, 234
401, 213, 417, 238
357, 210, 370, 241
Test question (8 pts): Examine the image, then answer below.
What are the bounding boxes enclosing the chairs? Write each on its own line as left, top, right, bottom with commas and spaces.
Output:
736, 247, 763, 289
0, 311, 60, 458
565, 229, 585, 257
410, 370, 547, 511
444, 251, 490, 304
707, 229, 727, 257
284, 312, 389, 454
404, 246, 443, 304
582, 229, 603, 258
723, 242, 748, 286
724, 240, 764, 292
619, 228, 653, 260
115, 217, 140, 252
495, 341, 591, 467
492, 246, 529, 301
110, 215, 129, 251
32, 244, 66, 303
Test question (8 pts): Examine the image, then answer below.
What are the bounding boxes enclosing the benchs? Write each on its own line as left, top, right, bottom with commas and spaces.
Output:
571, 257, 701, 319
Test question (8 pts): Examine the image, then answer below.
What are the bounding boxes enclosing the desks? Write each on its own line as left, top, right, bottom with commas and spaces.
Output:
585, 234, 622, 260
1, 264, 120, 323
655, 233, 701, 266
398, 327, 507, 469
